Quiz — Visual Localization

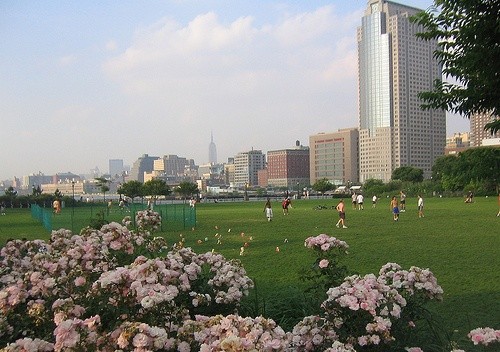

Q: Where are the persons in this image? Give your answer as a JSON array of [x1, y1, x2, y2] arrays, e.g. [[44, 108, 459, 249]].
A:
[[357, 193, 363, 210], [352, 192, 357, 208], [53, 200, 60, 212], [418, 195, 424, 218], [282, 198, 292, 216], [391, 196, 399, 222], [108, 199, 112, 208], [119, 198, 130, 212], [372, 194, 377, 208], [263, 198, 273, 221], [336, 199, 347, 228], [400, 190, 406, 212], [465, 191, 473, 203]]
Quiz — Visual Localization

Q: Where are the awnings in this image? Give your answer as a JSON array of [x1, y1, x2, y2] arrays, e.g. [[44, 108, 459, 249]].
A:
[[338, 186, 346, 189], [350, 186, 361, 189]]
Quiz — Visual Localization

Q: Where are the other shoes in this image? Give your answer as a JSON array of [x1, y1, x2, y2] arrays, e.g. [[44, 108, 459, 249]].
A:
[[336, 224, 339, 228], [343, 225, 348, 229]]
